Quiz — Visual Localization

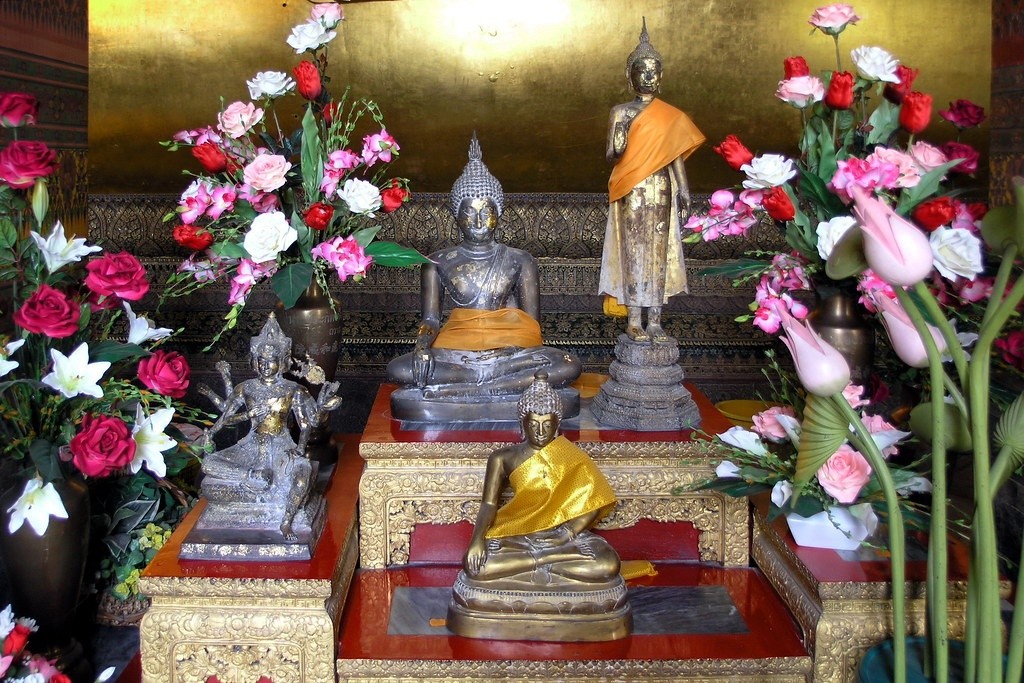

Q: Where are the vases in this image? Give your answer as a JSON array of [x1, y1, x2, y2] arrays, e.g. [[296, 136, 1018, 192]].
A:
[[5, 91, 225, 683], [172, 3, 447, 449], [676, 4, 995, 398]]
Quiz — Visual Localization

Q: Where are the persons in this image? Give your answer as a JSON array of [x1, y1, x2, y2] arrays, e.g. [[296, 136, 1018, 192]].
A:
[[461, 365, 624, 589], [196, 308, 342, 542], [383, 128, 582, 400], [597, 16, 706, 344]]
